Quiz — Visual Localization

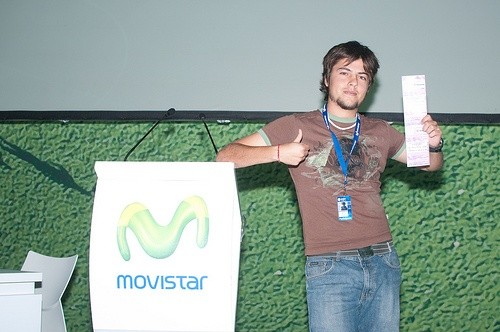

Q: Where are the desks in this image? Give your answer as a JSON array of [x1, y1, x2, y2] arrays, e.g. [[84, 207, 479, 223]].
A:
[[0, 269, 43, 332]]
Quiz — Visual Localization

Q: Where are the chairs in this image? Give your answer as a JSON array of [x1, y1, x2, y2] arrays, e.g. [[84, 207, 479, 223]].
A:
[[21, 251, 78, 332]]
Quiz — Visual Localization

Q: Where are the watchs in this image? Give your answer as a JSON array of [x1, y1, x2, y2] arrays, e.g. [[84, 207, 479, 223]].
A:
[[428, 138, 443, 152]]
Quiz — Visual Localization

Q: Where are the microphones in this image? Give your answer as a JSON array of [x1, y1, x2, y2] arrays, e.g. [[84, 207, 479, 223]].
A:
[[124, 108, 175, 161], [199, 113, 218, 155]]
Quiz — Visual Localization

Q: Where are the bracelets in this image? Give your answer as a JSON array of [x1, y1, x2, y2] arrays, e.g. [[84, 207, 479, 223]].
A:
[[278, 144, 280, 162]]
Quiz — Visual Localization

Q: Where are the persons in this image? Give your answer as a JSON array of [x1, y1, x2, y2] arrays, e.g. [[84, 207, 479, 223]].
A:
[[215, 40, 444, 331]]
